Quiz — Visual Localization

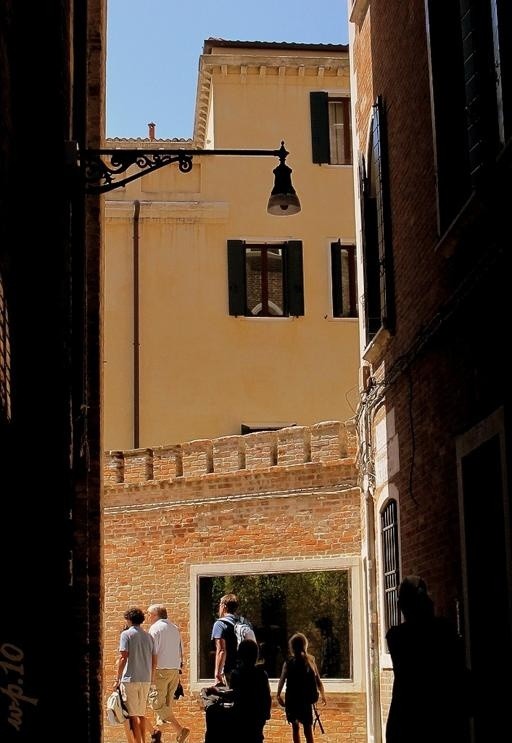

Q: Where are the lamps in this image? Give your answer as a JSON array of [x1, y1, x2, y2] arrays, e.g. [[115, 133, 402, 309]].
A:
[[73, 140, 301, 217]]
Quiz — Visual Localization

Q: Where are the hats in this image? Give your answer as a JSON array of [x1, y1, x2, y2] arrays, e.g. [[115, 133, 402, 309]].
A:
[[398, 574, 428, 599]]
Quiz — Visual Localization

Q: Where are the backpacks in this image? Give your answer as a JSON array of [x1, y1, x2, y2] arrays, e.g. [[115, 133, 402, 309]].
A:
[[285, 655, 319, 707], [215, 614, 259, 659]]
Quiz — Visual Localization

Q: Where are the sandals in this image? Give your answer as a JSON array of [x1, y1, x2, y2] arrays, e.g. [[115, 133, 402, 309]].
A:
[[150, 729, 162, 743], [175, 726, 191, 743]]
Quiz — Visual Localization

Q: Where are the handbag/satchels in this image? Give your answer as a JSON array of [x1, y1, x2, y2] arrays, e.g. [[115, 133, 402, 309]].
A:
[[105, 690, 129, 726], [173, 683, 184, 700]]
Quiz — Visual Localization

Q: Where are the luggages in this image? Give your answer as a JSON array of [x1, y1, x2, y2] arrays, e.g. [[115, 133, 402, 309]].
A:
[[203, 687, 257, 743]]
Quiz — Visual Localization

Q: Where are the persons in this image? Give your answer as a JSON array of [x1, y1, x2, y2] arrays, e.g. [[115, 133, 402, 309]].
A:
[[384, 574, 472, 743], [209, 592, 255, 688], [144, 602, 191, 742], [275, 632, 329, 743], [250, 622, 276, 678], [204, 638, 274, 743], [313, 615, 342, 678], [115, 622, 143, 743], [112, 606, 158, 743], [269, 623, 286, 678]]
